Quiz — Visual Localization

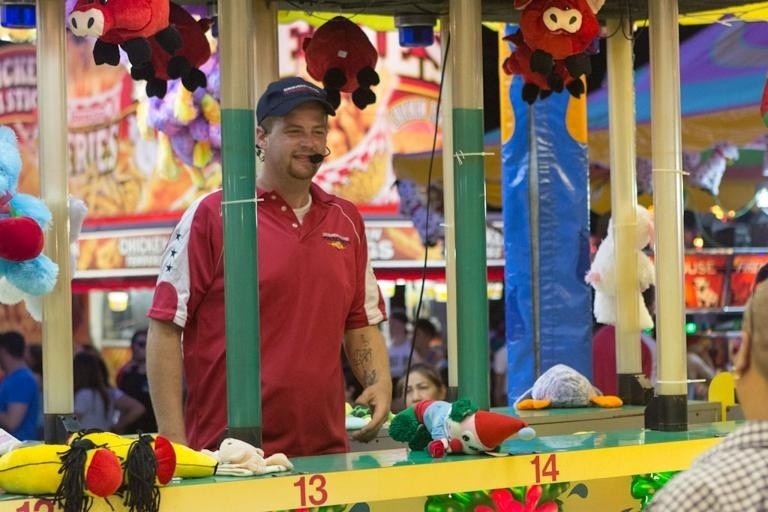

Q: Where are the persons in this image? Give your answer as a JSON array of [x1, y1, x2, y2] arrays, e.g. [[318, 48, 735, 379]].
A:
[[144, 77, 393, 457], [646, 284, 768, 512], [593, 284, 657, 400], [70, 352, 145, 436], [493, 348, 508, 407], [117, 330, 158, 435], [344, 366, 365, 405], [397, 364, 447, 410], [387, 311, 443, 379], [0, 331, 46, 441]]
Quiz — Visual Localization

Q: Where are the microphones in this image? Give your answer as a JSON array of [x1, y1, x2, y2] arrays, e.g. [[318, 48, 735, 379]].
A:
[[310, 146, 331, 164]]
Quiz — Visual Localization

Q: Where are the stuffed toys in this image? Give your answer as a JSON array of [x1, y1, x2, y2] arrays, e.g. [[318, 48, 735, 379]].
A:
[[584, 203, 654, 328], [0, 127, 58, 296], [147, 53, 221, 169], [394, 179, 445, 246], [388, 397, 536, 458], [66, 0, 214, 98], [0, 430, 294, 512], [512, 364, 624, 411], [636, 144, 742, 196], [502, 0, 606, 106], [302, 16, 381, 111]]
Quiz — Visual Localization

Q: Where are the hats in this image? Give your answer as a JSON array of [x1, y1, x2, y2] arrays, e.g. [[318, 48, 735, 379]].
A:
[[257, 77, 335, 125]]
